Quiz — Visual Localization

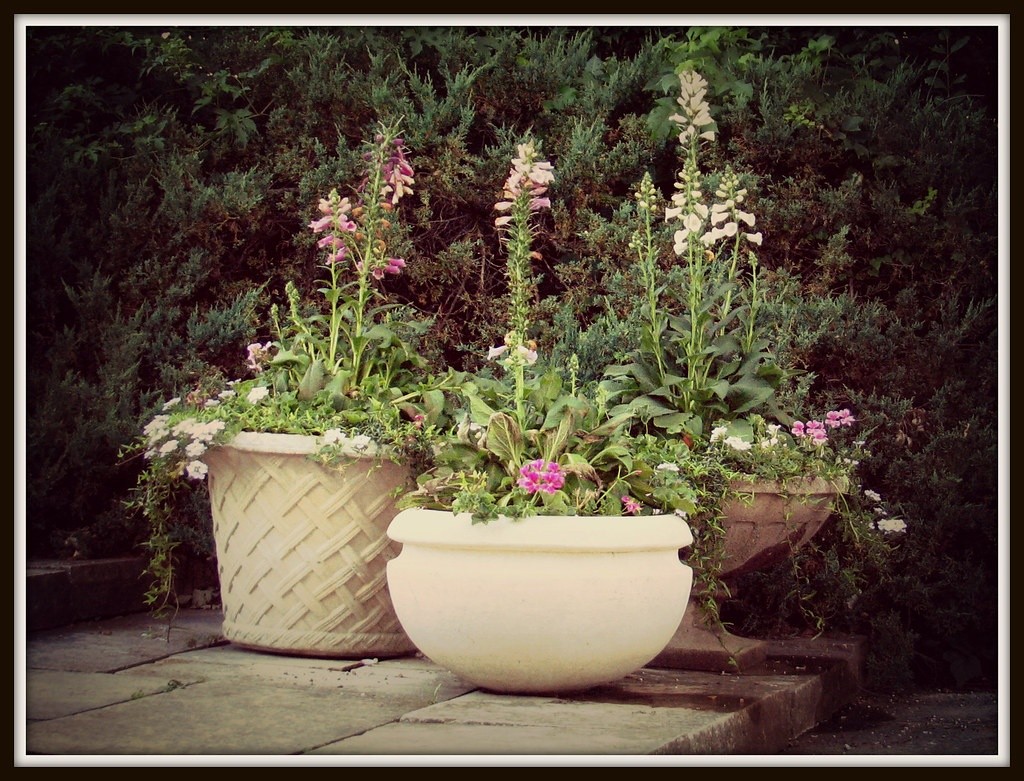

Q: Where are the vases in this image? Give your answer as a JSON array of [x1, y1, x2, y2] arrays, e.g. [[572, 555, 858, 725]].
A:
[[386, 509, 694, 694], [203, 430, 421, 656], [655, 473, 852, 670]]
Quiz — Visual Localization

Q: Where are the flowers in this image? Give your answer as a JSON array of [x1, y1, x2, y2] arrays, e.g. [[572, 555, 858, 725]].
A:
[[117, 62, 912, 647]]
